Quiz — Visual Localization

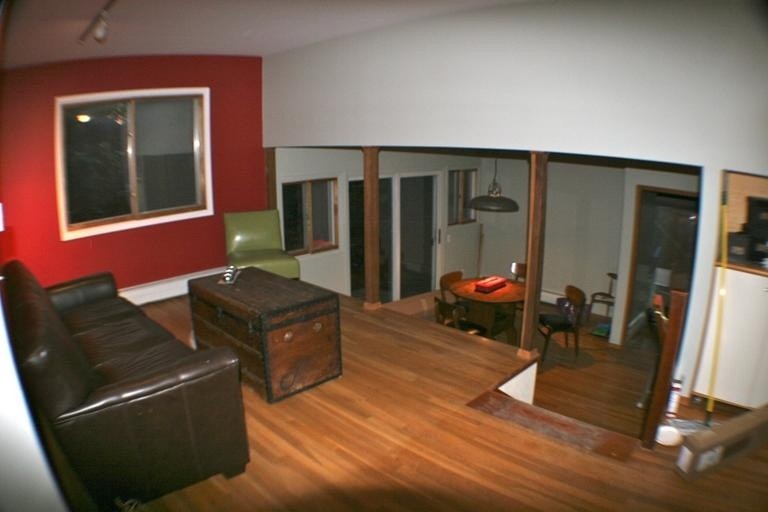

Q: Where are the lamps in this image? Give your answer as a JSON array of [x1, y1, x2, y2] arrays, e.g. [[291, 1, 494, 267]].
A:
[[653, 268, 672, 295], [466, 157, 520, 213]]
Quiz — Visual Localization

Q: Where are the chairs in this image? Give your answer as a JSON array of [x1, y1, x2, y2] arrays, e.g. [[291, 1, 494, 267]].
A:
[[223, 209, 300, 281], [433, 262, 585, 367], [586, 272, 618, 329]]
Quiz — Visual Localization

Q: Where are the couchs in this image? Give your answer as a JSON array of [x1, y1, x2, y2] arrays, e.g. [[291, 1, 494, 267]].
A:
[[0, 259, 252, 512]]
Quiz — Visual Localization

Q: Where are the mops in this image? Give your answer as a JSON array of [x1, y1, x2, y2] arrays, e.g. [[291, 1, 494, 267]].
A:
[[666, 188, 729, 438]]
